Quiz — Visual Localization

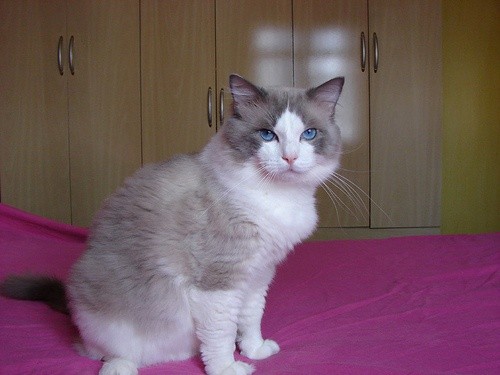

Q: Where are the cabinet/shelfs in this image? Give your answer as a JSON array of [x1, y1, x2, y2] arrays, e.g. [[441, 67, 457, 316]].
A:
[[0, 0, 443, 242]]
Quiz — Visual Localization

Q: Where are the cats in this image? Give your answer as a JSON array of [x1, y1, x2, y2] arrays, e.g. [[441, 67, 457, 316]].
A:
[[1, 70, 395, 375]]
